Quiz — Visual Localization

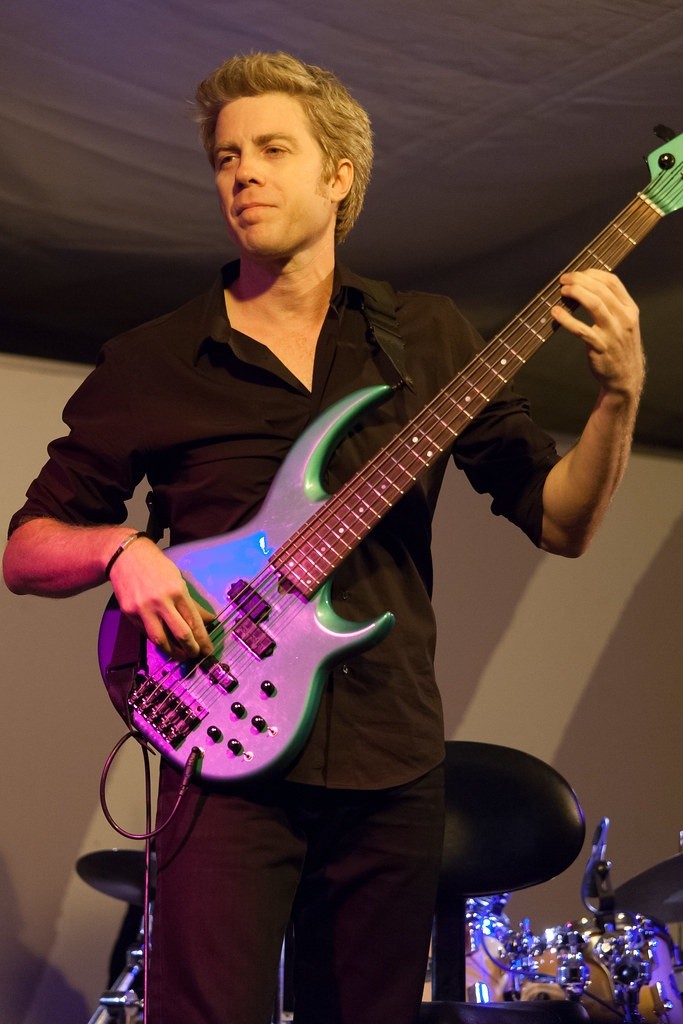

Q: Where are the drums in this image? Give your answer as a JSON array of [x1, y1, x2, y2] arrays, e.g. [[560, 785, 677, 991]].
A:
[[420, 910, 522, 1003], [577, 911, 683, 1024], [513, 924, 638, 1015]]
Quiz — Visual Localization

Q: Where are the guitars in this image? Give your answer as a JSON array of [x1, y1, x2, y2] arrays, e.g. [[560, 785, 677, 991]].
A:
[[93, 129, 683, 787]]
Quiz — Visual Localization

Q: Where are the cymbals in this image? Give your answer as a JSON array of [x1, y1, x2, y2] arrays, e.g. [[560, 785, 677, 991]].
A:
[[596, 852, 683, 923], [437, 741, 586, 898], [75, 850, 156, 907]]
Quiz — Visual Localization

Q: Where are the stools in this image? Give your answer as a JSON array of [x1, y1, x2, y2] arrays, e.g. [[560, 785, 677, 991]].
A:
[[413, 739, 591, 1024]]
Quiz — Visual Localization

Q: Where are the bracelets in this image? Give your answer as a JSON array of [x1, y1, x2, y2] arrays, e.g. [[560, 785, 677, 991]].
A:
[[105, 531, 152, 581]]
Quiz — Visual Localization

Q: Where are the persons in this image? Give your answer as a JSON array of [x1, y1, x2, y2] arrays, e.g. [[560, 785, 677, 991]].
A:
[[4, 54, 645, 1024]]
